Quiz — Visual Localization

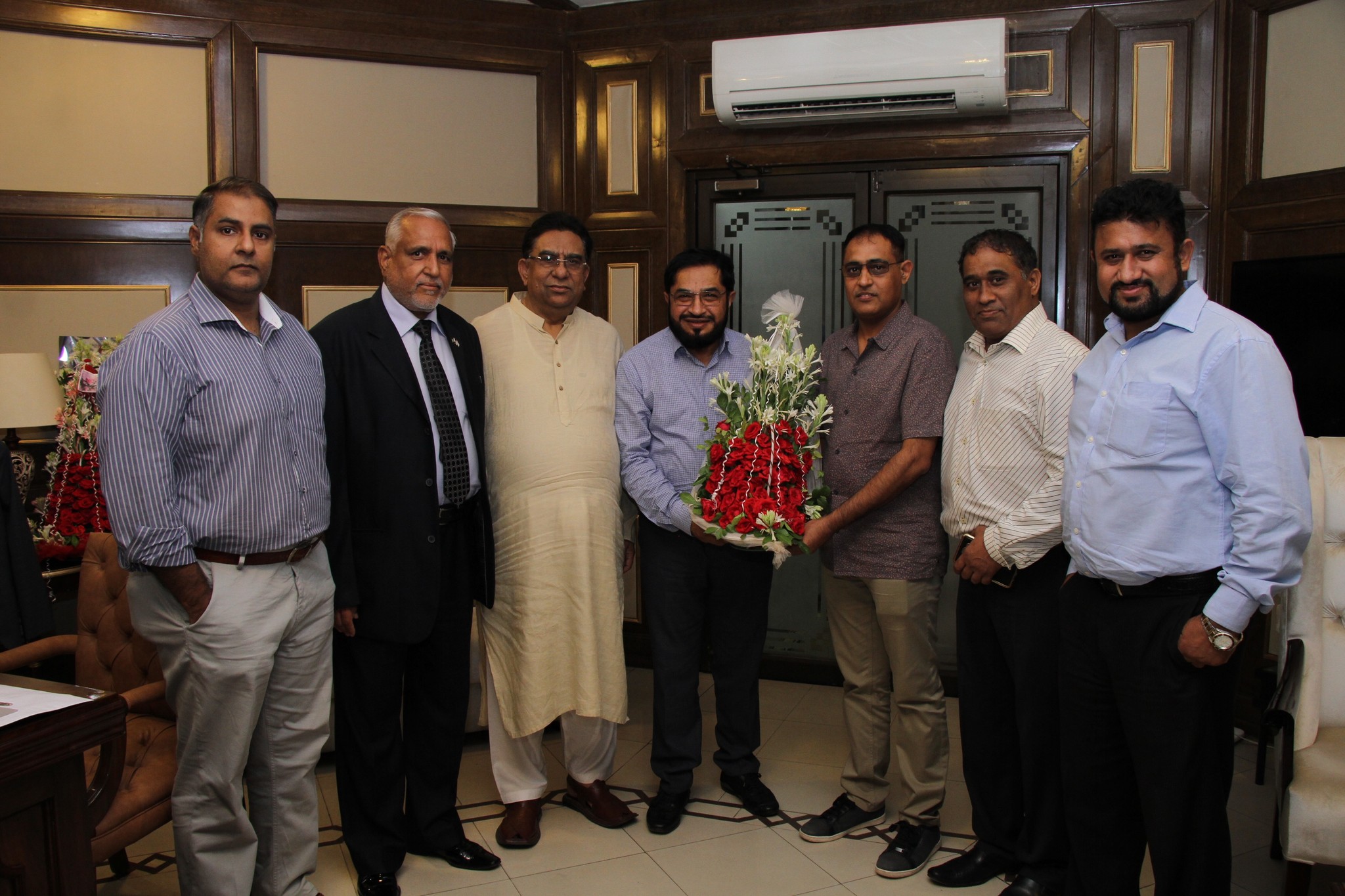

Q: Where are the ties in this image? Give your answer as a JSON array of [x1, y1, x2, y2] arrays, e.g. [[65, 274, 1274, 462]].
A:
[[412, 320, 471, 508]]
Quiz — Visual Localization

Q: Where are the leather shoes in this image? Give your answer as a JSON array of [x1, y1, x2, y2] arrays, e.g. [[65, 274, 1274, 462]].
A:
[[357, 867, 401, 896], [563, 773, 639, 828], [496, 797, 542, 845], [927, 841, 1006, 887], [646, 779, 691, 834], [438, 839, 501, 871], [997, 873, 1045, 896], [720, 771, 779, 817]]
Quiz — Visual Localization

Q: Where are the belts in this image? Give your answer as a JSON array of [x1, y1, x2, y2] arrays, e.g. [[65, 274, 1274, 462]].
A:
[[439, 499, 480, 526], [1092, 569, 1221, 600], [192, 533, 323, 565]]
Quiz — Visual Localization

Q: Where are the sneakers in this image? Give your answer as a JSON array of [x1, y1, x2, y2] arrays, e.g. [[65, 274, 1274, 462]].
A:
[[875, 820, 942, 878], [799, 792, 886, 843]]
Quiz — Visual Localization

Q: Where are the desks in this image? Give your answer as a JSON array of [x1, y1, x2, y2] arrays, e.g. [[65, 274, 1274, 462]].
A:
[[0, 671, 121, 896]]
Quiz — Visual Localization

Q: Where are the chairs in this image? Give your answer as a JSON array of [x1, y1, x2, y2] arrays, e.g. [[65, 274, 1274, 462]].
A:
[[0, 532, 177, 881], [1262, 435, 1344, 896]]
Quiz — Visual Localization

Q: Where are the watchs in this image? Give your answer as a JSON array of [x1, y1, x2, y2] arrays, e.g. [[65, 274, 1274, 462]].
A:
[[1198, 610, 1243, 652]]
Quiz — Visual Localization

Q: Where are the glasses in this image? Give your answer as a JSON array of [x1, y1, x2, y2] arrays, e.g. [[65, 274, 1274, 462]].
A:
[[670, 291, 727, 305], [528, 254, 588, 270], [840, 260, 901, 278]]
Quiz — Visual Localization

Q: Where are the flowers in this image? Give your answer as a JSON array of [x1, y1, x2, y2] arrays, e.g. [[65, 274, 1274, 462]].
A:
[[677, 289, 835, 569], [23, 333, 126, 559]]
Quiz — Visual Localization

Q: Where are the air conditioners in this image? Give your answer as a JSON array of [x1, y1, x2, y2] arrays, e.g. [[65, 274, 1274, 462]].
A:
[[712, 18, 1010, 128]]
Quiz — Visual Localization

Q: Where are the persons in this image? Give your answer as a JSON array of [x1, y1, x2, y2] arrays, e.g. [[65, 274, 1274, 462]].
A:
[[293, 210, 503, 896], [615, 244, 788, 834], [1053, 178, 1315, 896], [922, 230, 1091, 896], [788, 223, 956, 879], [95, 176, 338, 896], [465, 213, 638, 850]]
